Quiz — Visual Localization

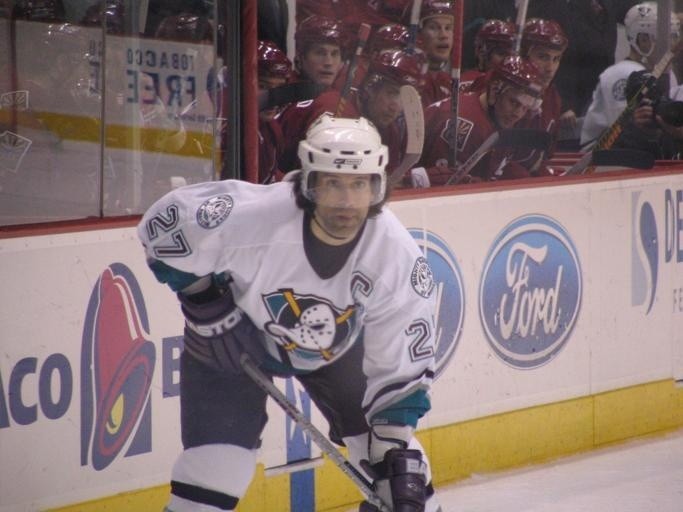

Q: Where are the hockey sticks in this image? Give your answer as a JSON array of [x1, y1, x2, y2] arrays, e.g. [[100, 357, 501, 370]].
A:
[[561, 151, 656, 178], [447, 129, 556, 183]]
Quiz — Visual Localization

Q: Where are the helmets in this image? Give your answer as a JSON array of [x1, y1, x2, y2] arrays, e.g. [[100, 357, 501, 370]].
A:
[[258, 38, 293, 74], [494, 57, 545, 99], [18, 0, 209, 121], [624, 0, 683, 57], [363, 50, 420, 88], [360, 22, 411, 49], [296, 13, 346, 54], [474, 18, 515, 59], [412, 1, 456, 30], [297, 110, 389, 208], [520, 16, 568, 57]]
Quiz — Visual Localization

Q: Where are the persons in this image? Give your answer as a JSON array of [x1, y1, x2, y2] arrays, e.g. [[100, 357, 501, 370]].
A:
[[134, 110, 443, 511], [0, 1, 682, 225]]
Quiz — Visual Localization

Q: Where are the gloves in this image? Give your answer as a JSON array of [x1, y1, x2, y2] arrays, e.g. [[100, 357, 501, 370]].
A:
[[172, 287, 265, 376], [264, 78, 328, 107], [352, 442, 430, 511]]
